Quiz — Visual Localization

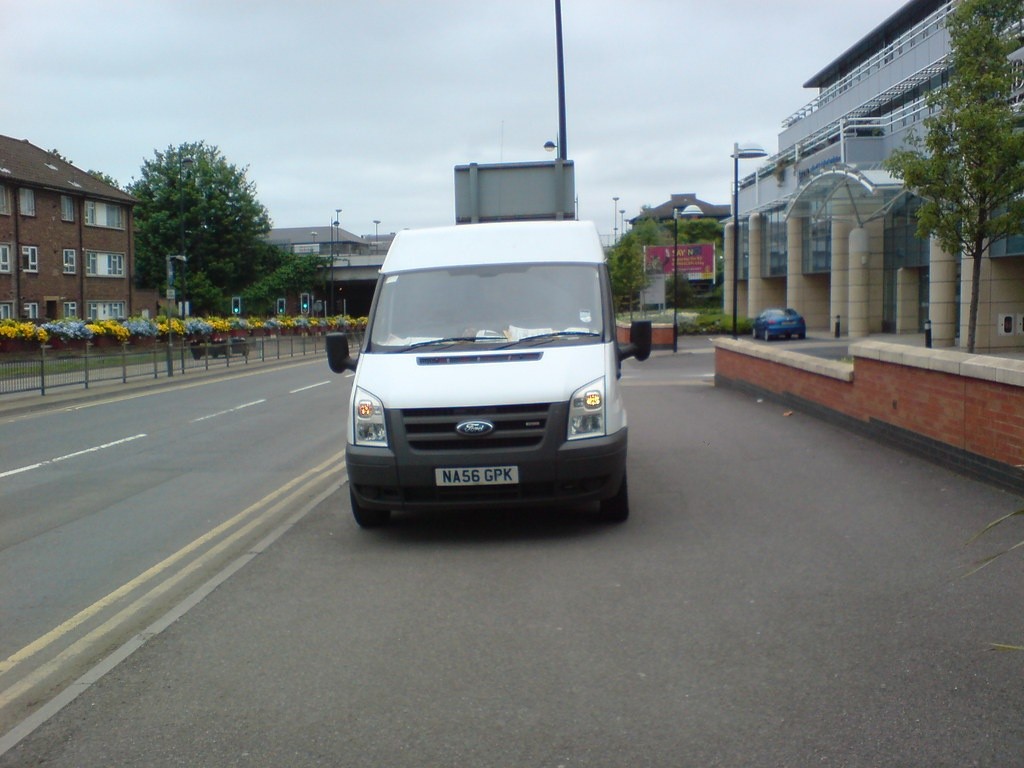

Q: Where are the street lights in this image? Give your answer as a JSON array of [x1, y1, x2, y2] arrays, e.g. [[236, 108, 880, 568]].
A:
[[729, 140, 768, 338], [330, 217, 340, 316], [612, 196, 619, 245], [166, 253, 187, 376], [673, 204, 704, 355], [624, 219, 629, 232], [619, 209, 626, 234], [373, 219, 381, 255], [544, 134, 567, 160], [336, 209, 342, 256]]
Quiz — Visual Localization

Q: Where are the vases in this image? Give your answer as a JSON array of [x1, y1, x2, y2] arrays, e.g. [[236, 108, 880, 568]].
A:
[[23, 336, 42, 351], [231, 328, 249, 337], [160, 332, 178, 343], [320, 326, 330, 333], [94, 334, 124, 347], [210, 332, 228, 344], [280, 326, 295, 336], [188, 331, 203, 341], [336, 325, 345, 332], [296, 327, 311, 337], [50, 334, 90, 350], [266, 328, 278, 335], [251, 327, 266, 336], [127, 333, 157, 348], [309, 327, 321, 335], [0, 337, 24, 353]]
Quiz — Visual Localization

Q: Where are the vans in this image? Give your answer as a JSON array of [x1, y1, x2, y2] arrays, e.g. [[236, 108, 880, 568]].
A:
[[323, 220, 652, 526]]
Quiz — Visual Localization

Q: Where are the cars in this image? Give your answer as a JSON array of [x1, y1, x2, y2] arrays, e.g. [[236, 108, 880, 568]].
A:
[[756, 307, 806, 342]]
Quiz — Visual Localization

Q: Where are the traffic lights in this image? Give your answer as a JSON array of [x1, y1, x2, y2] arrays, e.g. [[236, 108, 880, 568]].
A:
[[299, 292, 310, 315], [277, 299, 286, 315], [232, 296, 240, 315]]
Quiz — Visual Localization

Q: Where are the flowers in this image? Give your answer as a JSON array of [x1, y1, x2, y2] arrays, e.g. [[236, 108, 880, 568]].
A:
[[0, 313, 368, 349]]
